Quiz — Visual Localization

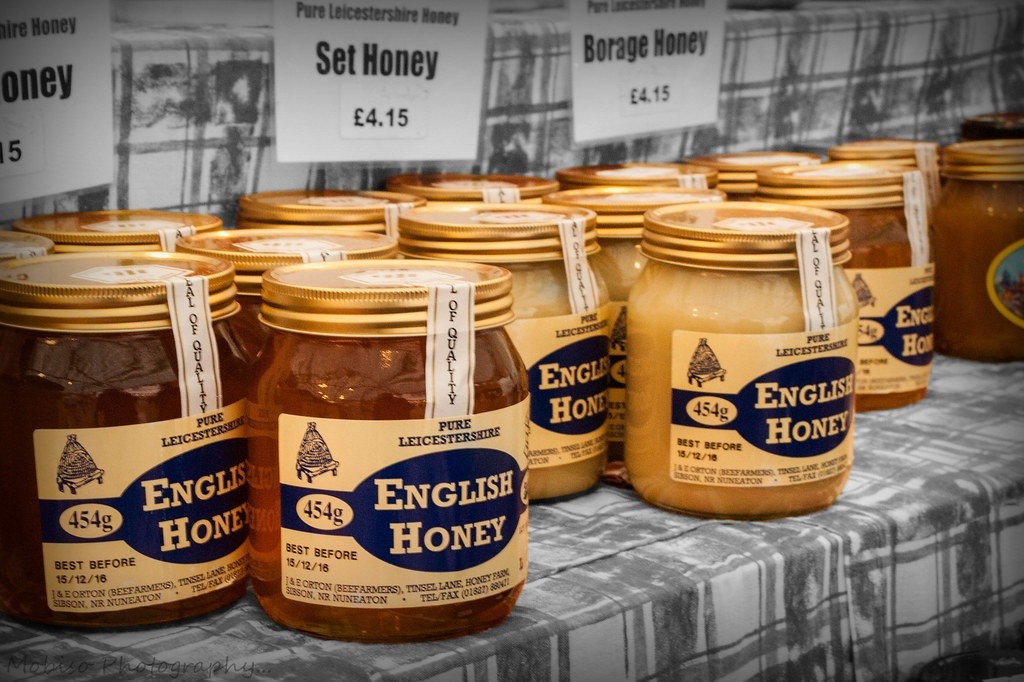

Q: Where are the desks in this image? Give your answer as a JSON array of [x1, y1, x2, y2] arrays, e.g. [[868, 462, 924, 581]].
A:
[[2, 1, 1023, 681]]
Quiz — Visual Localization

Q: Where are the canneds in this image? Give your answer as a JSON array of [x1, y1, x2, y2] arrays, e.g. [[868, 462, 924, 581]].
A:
[[4, 115, 1021, 644]]
